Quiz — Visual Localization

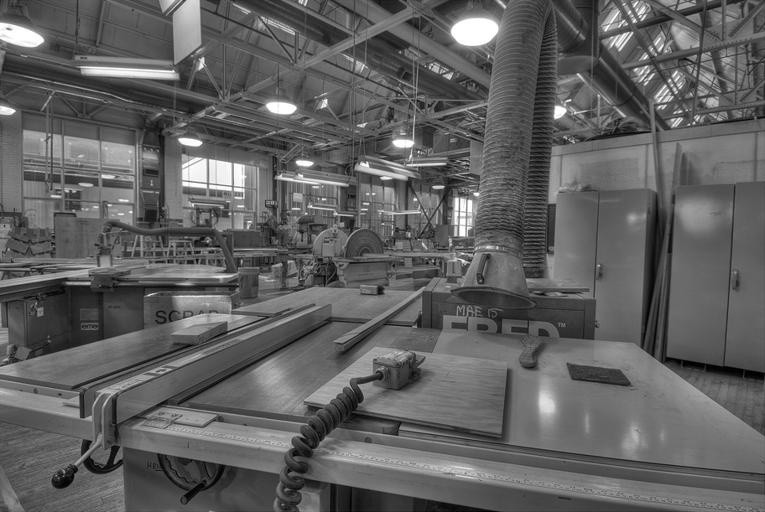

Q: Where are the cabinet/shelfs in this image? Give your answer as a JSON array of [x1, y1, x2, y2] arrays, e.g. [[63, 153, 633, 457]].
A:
[[665, 181, 765, 378], [552, 188, 657, 347]]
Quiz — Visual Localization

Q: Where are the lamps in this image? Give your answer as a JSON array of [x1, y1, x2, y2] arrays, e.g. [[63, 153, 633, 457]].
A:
[[0, 0, 45, 49], [391, 128, 415, 149], [448, 0, 503, 47], [265, 86, 298, 116]]
